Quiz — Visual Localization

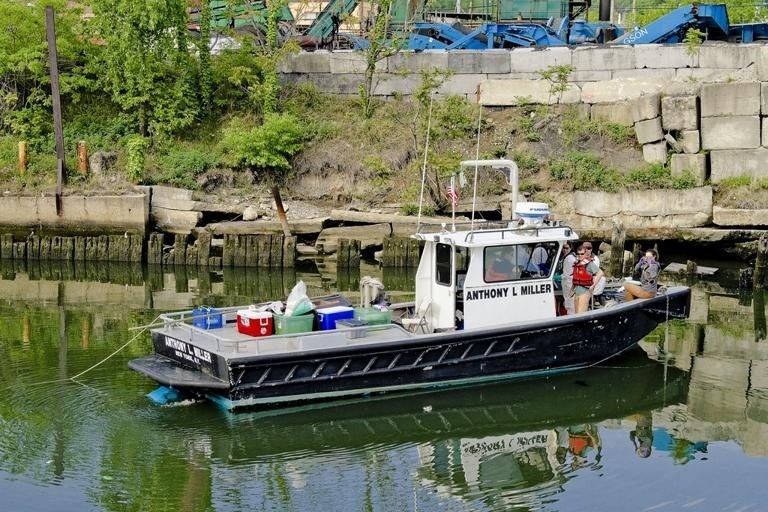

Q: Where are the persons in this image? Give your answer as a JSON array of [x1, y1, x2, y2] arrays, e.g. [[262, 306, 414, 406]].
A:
[[622, 409, 653, 459], [483, 239, 607, 315], [624, 248, 661, 302], [553, 418, 602, 472]]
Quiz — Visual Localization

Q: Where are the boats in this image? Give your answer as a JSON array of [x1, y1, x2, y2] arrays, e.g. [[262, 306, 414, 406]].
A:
[[175, 358, 697, 512], [125, 91, 692, 423]]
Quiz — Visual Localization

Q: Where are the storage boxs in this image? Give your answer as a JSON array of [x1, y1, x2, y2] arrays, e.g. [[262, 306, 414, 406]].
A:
[[236, 305, 354, 337]]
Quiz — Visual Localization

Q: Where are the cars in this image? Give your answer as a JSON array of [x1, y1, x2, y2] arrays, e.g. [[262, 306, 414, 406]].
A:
[[143, 25, 242, 63]]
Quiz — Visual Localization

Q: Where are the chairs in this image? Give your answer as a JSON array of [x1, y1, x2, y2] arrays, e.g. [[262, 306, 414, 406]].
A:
[[402, 295, 433, 334]]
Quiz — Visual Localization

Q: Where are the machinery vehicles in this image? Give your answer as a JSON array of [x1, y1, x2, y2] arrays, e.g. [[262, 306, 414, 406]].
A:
[[186, 0, 361, 49]]
